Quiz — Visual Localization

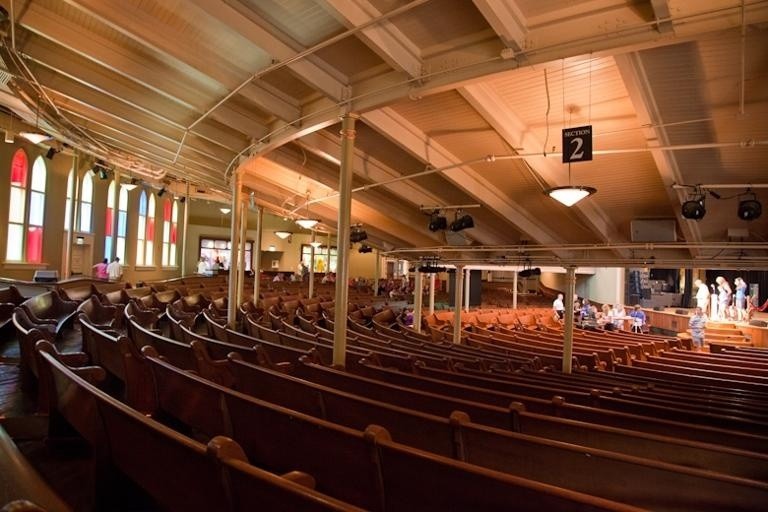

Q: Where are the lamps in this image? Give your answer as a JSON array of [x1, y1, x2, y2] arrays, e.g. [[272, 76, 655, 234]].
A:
[[273, 215, 294, 241], [120, 161, 137, 193], [294, 191, 321, 231], [541, 151, 597, 209], [220, 190, 235, 218], [19, 94, 55, 147]]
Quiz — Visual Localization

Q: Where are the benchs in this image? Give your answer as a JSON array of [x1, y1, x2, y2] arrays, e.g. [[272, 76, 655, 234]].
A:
[[0, 266, 766, 512]]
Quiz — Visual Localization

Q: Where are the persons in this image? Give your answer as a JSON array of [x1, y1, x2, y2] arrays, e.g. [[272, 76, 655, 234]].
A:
[[573, 295, 579, 302], [275, 262, 311, 282], [92, 258, 108, 281], [197, 257, 210, 275], [630, 304, 646, 325], [215, 256, 220, 270], [589, 305, 599, 314], [322, 272, 441, 300], [601, 304, 612, 323], [694, 279, 710, 315], [405, 309, 414, 325], [553, 294, 566, 318], [574, 301, 581, 321], [580, 298, 591, 318], [734, 278, 749, 321], [401, 307, 409, 321], [612, 304, 625, 329], [106, 257, 123, 282], [715, 276, 732, 321], [688, 307, 707, 347]]
